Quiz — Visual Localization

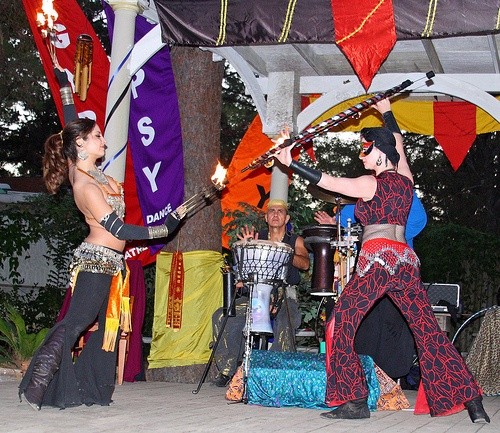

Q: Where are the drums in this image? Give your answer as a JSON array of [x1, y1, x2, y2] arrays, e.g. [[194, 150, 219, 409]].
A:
[[230, 239, 295, 335], [303, 224, 341, 298]]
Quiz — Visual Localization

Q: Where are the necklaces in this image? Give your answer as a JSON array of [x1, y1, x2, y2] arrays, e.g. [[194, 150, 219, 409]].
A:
[[387, 169, 397, 172], [267, 232, 284, 242], [84, 166, 109, 184]]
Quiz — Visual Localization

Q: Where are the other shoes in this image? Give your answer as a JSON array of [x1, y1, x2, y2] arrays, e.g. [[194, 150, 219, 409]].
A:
[[216, 374, 230, 386], [463, 394, 490, 424], [320, 399, 370, 419]]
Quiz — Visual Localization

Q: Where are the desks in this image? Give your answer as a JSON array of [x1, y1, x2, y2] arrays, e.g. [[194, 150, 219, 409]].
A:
[[434, 313, 470, 340]]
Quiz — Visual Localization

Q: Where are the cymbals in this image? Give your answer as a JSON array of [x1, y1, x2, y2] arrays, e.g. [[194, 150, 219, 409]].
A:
[[305, 182, 358, 206]]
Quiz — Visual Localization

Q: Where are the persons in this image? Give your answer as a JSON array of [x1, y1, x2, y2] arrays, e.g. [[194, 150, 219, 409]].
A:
[[18, 42, 187, 412], [212, 199, 310, 387], [314, 189, 427, 380], [267, 93, 490, 424]]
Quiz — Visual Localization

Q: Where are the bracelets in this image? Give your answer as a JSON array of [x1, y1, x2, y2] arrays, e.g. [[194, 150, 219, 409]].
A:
[[147, 223, 168, 239]]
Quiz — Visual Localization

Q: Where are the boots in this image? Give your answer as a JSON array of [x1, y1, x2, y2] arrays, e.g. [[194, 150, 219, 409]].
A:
[[23, 325, 65, 411]]
